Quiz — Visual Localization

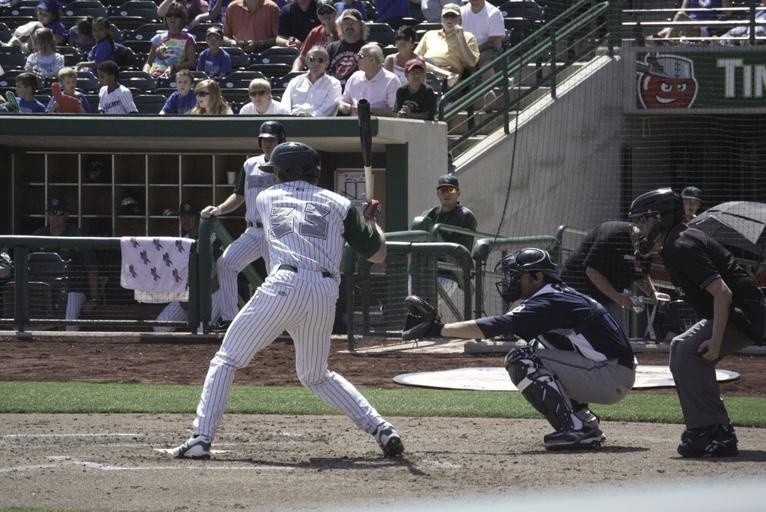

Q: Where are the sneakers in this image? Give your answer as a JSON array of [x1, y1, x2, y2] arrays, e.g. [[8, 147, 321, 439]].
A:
[[171, 436, 211, 459], [678, 422, 738, 457], [208, 316, 231, 332], [544, 407, 606, 451], [374, 421, 404, 456]]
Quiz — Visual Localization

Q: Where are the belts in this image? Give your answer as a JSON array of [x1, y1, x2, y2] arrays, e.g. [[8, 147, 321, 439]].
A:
[[277, 263, 332, 277], [245, 222, 262, 227]]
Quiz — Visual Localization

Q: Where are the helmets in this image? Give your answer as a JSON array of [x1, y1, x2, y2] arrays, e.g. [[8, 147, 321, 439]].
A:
[[258, 121, 285, 143], [495, 248, 558, 301], [626, 187, 684, 259], [259, 142, 321, 183]]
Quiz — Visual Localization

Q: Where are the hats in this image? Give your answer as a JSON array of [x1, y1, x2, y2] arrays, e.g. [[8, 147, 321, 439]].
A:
[[179, 202, 200, 217], [317, 0, 336, 15], [47, 199, 67, 212], [442, 4, 460, 17], [436, 175, 458, 190], [405, 60, 425, 73], [682, 185, 703, 202], [206, 26, 222, 38]]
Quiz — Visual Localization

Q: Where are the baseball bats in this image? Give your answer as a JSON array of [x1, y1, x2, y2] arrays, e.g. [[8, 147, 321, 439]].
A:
[[357, 99, 374, 202]]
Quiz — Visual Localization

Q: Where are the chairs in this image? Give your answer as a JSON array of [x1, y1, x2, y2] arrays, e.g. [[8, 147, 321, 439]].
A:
[[0, 1, 549, 115]]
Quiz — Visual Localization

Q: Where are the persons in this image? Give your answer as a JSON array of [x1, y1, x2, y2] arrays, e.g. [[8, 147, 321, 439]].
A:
[[407, 175, 478, 306], [402, 247, 639, 449], [643, 0, 766, 46], [171, 141, 404, 457], [0, 196, 225, 331], [200, 120, 285, 333], [0, 0, 547, 120], [560, 221, 656, 310], [654, 185, 705, 344], [628, 187, 766, 456]]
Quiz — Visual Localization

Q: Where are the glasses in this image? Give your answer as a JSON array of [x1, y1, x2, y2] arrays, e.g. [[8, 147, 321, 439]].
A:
[[195, 90, 208, 97], [309, 56, 322, 62], [165, 12, 181, 18], [47, 211, 64, 216], [250, 90, 265, 96]]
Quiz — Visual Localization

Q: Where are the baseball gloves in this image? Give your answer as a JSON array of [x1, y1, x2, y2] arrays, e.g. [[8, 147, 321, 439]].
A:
[[402, 295, 445, 340]]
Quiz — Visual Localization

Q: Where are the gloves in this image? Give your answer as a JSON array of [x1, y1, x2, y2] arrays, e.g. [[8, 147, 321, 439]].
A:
[[361, 200, 381, 221]]
